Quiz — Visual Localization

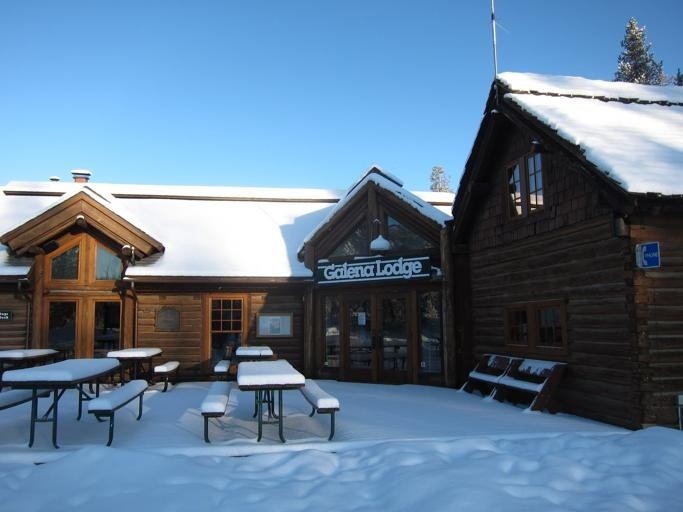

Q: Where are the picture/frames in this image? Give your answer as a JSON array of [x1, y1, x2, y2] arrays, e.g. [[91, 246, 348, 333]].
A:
[[255, 312, 294, 338]]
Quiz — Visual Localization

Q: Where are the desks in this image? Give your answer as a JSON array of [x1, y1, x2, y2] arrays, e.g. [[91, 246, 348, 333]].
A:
[[0, 348, 163, 449], [235, 346, 305, 443]]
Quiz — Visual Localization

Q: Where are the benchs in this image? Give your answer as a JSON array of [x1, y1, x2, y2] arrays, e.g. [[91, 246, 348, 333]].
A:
[[454, 353, 568, 415], [300, 378, 341, 440], [88, 362, 182, 446], [201, 359, 232, 443]]
